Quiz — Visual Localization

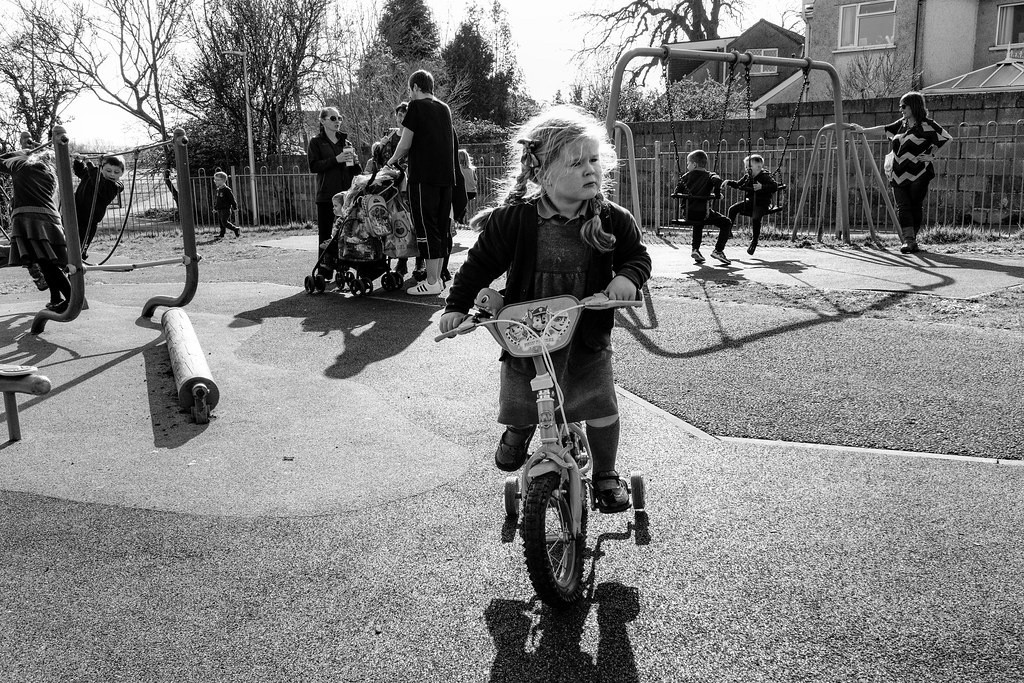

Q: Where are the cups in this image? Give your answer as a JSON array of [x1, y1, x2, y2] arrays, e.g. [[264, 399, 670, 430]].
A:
[[343, 146, 355, 167]]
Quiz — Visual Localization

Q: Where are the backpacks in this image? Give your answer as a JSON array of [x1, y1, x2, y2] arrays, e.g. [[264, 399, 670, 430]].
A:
[[355, 195, 393, 240]]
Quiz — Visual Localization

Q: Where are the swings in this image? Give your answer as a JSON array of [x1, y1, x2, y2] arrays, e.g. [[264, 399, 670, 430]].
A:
[[659, 44, 741, 225], [739, 51, 813, 218]]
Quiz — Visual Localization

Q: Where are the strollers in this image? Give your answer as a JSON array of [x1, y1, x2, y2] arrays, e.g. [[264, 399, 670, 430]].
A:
[[304, 158, 406, 299]]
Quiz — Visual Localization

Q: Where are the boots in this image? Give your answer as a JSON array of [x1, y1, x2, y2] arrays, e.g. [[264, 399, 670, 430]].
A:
[[899, 226, 919, 254]]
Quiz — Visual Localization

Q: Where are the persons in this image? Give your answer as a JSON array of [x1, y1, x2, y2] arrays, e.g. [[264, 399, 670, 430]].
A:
[[0, 149, 70, 314], [438, 103, 654, 513], [306, 107, 362, 280], [721, 154, 778, 255], [317, 191, 348, 250], [385, 68, 457, 296], [212, 172, 241, 239], [673, 149, 733, 265], [379, 101, 426, 276], [456, 149, 478, 199], [849, 92, 953, 253], [27, 152, 126, 291], [363, 141, 381, 175]]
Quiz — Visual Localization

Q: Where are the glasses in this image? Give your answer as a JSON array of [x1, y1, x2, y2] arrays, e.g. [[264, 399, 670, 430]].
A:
[[325, 115, 343, 122], [898, 104, 907, 110]]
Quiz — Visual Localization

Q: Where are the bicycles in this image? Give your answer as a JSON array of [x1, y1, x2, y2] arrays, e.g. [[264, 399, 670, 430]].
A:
[[434, 287, 646, 605]]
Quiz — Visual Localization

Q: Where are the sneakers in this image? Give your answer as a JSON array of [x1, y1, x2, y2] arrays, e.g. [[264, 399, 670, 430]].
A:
[[406, 278, 445, 295]]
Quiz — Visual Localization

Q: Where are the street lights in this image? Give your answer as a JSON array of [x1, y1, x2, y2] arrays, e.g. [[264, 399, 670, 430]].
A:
[[221, 50, 258, 228]]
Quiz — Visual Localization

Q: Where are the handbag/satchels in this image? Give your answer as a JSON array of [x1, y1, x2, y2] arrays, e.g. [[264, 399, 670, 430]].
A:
[[883, 150, 894, 180], [383, 198, 420, 258]]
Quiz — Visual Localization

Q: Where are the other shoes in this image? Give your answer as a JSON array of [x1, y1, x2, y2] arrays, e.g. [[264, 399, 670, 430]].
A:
[[395, 261, 408, 276], [415, 269, 452, 281], [690, 248, 706, 262], [715, 230, 733, 240], [591, 469, 629, 513], [495, 425, 537, 471], [32, 271, 49, 291], [412, 262, 425, 277], [709, 249, 732, 264], [45, 297, 90, 313], [235, 227, 240, 238], [747, 241, 757, 255]]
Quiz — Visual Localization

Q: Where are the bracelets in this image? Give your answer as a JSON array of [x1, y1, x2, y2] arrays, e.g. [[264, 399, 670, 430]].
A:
[[932, 153, 936, 160]]
